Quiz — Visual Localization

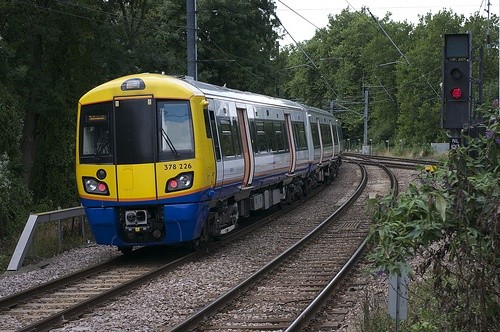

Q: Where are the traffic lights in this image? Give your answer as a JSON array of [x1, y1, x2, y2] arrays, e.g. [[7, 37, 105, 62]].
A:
[[440, 33, 473, 129]]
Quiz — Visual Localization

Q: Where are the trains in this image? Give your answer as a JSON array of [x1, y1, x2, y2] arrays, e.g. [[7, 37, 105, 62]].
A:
[[75, 71, 343, 257]]
